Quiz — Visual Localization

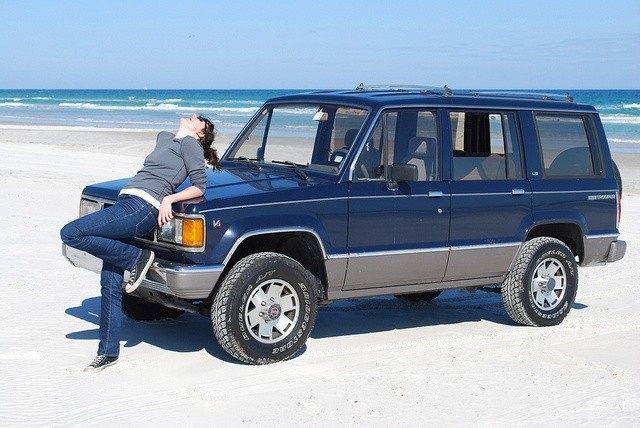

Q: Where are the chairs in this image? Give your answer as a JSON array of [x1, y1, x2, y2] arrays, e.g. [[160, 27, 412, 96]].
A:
[[342, 129, 380, 176], [402, 137, 437, 183]]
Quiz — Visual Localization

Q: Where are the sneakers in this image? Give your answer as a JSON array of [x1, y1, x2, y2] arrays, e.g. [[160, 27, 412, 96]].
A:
[[83, 355, 120, 374], [125, 249, 155, 293]]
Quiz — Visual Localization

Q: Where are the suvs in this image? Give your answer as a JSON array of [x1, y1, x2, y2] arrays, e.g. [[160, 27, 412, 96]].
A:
[[63, 85, 627, 365]]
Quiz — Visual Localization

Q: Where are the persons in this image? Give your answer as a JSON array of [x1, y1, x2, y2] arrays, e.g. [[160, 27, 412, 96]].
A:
[[60, 113, 223, 373]]
